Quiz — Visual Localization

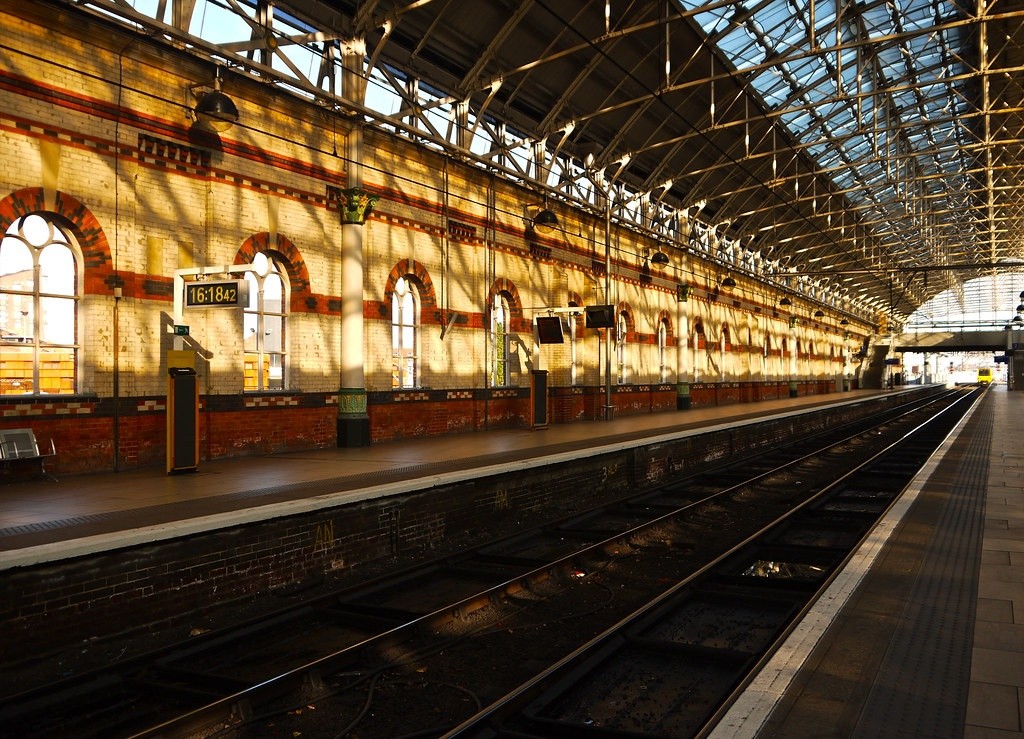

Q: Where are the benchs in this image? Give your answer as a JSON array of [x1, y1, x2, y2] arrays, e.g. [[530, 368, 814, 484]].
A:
[[0, 428, 61, 484]]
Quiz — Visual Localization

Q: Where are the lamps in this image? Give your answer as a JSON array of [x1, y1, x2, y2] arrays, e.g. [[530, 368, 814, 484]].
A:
[[1013, 314, 1023, 323], [776, 289, 792, 308], [836, 314, 850, 327], [1020, 291, 1024, 300], [716, 270, 736, 291], [188, 76, 241, 132], [1016, 303, 1024, 313], [644, 241, 669, 270], [810, 304, 824, 319], [524, 200, 560, 233]]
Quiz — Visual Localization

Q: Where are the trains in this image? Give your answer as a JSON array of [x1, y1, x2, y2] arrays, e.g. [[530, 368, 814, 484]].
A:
[[978, 368, 994, 386]]
